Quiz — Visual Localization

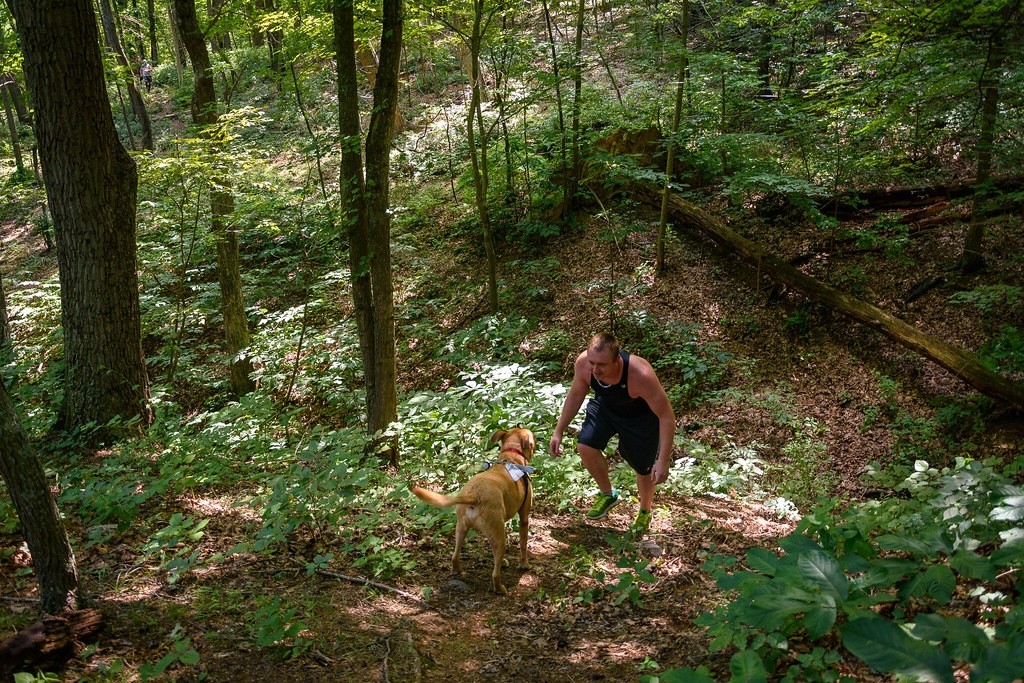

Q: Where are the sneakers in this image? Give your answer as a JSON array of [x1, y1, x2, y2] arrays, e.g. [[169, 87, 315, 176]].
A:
[[630, 509, 652, 536], [586, 488, 620, 520]]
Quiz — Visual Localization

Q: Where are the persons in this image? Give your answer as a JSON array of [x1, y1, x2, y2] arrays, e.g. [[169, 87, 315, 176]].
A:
[[547, 333, 676, 533], [139, 60, 152, 93], [136, 68, 143, 84]]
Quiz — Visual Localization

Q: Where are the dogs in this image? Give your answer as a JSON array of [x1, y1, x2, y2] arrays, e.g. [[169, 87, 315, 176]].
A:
[[411, 428, 534, 595]]
